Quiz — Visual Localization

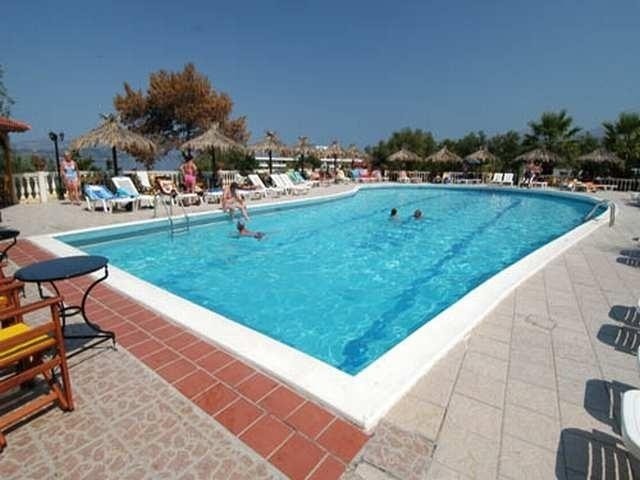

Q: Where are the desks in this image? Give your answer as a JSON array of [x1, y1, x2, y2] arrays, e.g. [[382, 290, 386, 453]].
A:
[[0, 225, 19, 261], [575, 183, 592, 192], [14, 256, 115, 377]]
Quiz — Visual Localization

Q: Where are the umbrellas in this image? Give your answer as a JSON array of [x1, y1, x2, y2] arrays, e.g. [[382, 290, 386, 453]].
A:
[[463, 149, 497, 178], [343, 144, 360, 167], [316, 139, 347, 170], [279, 135, 321, 177], [577, 146, 621, 167], [67, 115, 157, 176], [386, 147, 425, 169], [425, 145, 463, 164], [180, 127, 246, 188], [512, 146, 561, 164], [245, 132, 295, 184]]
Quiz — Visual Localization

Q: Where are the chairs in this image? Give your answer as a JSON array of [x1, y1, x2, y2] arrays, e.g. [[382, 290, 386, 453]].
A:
[[0, 281, 74, 453], [0, 261, 17, 324]]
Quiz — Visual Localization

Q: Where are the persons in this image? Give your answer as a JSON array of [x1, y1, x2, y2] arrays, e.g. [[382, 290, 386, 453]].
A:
[[234, 174, 257, 190], [60, 150, 82, 205], [211, 161, 224, 192], [221, 181, 250, 220], [389, 208, 399, 219], [235, 220, 256, 235], [178, 154, 198, 193], [414, 209, 421, 218]]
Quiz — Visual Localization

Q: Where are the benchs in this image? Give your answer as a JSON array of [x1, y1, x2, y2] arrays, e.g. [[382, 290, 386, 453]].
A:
[[518, 181, 547, 189], [594, 185, 617, 191]]
